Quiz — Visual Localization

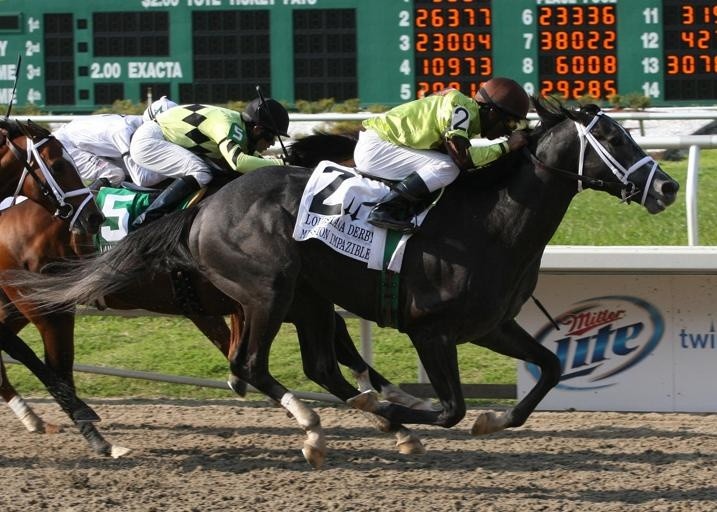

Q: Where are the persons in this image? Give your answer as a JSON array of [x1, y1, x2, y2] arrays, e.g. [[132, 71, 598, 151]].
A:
[[129, 98, 291, 228], [354, 77, 529, 233], [53, 96, 178, 190]]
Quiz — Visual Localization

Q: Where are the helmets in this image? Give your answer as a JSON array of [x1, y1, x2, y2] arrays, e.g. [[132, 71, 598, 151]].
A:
[[475, 78, 533, 124], [143, 96, 179, 124]]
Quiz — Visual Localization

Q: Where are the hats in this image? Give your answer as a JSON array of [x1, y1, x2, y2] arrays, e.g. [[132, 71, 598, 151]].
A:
[[246, 98, 289, 138]]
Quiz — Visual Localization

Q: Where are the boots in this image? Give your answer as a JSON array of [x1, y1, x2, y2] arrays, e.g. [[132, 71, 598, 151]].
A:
[[87, 176, 112, 192], [368, 172, 429, 233], [132, 175, 201, 229]]
